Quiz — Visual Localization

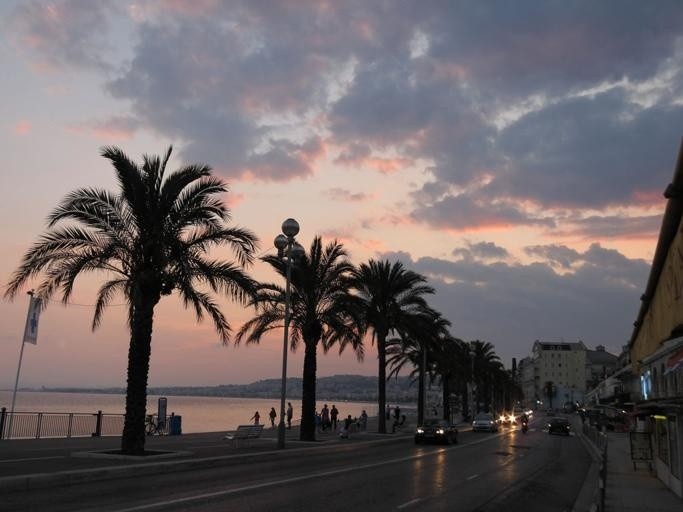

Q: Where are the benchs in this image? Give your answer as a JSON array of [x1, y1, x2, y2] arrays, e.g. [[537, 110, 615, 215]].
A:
[[221, 424, 264, 450]]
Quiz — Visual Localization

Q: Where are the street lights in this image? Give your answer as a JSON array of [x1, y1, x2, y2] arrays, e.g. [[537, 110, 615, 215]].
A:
[[274, 218, 300, 448]]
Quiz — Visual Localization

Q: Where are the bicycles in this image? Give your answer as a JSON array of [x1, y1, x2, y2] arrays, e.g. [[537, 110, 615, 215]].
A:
[[145, 412, 175, 436]]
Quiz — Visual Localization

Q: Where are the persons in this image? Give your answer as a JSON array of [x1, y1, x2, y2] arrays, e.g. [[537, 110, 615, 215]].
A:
[[432, 406, 438, 416], [395, 405, 400, 422], [249, 411, 261, 425], [387, 404, 391, 419], [287, 402, 294, 429], [269, 408, 277, 427], [321, 404, 368, 439], [521, 413, 528, 427]]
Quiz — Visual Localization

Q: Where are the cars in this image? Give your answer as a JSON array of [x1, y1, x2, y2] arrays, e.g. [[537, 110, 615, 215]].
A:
[[415, 417, 457, 445], [472, 414, 499, 434], [547, 416, 570, 436], [497, 404, 534, 426]]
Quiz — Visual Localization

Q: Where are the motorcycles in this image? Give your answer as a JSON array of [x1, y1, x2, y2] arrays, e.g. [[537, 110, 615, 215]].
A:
[[520, 415, 530, 434]]
